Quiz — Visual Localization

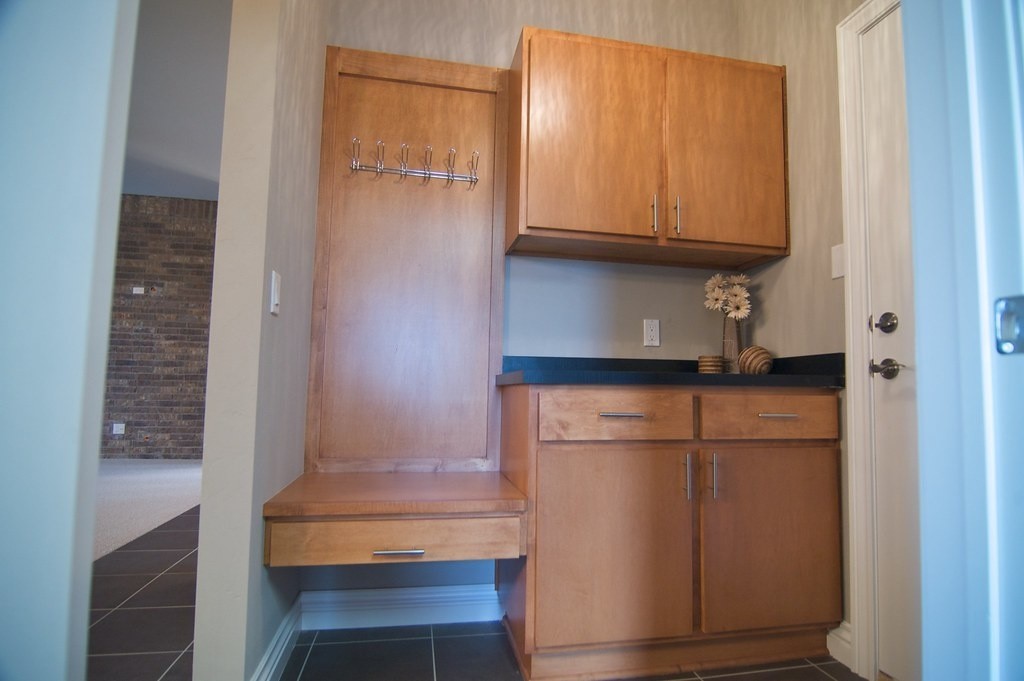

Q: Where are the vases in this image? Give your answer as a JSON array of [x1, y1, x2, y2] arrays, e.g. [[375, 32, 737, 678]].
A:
[[724, 316, 745, 374]]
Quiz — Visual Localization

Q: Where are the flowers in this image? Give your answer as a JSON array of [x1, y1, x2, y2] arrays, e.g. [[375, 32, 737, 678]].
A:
[[703, 273, 752, 320]]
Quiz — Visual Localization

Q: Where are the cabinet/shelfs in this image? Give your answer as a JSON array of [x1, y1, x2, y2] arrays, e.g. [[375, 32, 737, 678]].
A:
[[504, 24, 791, 272], [495, 384, 843, 681], [264, 470, 528, 566]]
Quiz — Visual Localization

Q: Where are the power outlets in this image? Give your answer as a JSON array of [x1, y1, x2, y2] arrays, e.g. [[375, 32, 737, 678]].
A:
[[643, 318, 660, 346]]
